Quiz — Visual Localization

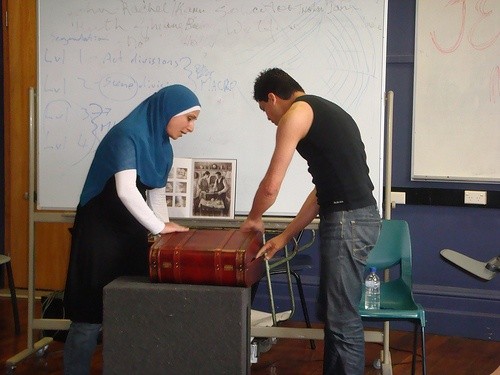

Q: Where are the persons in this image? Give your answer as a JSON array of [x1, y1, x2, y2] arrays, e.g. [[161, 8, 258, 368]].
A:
[[197, 171, 229, 203], [61, 85, 201, 375], [240, 68, 381, 375]]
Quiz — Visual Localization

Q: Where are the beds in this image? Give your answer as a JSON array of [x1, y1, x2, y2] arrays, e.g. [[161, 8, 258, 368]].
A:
[[249, 253, 317, 350]]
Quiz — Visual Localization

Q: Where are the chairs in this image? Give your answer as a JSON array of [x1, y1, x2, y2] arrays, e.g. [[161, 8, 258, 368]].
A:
[[0, 254, 21, 336], [356, 220, 427, 375]]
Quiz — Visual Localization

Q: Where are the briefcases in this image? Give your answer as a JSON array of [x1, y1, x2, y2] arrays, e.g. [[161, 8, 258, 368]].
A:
[[148, 227, 269, 288]]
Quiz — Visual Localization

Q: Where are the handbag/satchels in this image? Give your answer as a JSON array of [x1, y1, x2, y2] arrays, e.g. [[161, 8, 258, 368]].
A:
[[38, 292, 71, 343]]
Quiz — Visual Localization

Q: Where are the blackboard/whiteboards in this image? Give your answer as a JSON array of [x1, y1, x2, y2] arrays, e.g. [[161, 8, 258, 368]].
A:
[[409, 0, 500, 182], [36, 1, 389, 219]]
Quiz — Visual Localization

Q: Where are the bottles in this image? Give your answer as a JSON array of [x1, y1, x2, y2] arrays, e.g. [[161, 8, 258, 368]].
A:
[[364, 266, 381, 310]]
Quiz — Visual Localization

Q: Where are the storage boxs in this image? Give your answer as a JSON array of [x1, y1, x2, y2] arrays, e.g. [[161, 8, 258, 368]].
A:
[[146, 229, 266, 287]]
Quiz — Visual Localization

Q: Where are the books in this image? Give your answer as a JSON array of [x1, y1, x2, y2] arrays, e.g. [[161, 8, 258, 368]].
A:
[[164, 156, 237, 219]]
